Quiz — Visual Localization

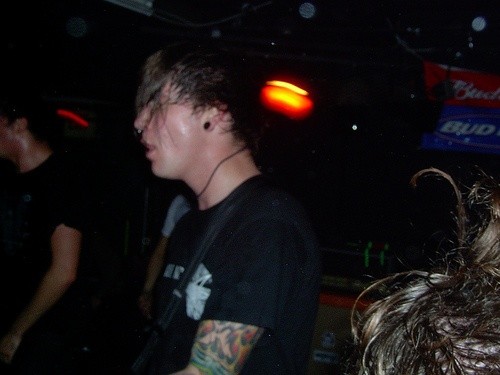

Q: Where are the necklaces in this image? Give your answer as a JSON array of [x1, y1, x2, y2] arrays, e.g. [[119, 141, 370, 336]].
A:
[[197, 144, 250, 198]]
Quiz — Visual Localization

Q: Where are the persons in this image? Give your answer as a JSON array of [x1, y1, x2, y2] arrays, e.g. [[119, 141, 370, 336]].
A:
[[138, 196, 192, 319], [338, 168, 500, 375], [0, 97, 88, 375], [127, 41, 320, 375]]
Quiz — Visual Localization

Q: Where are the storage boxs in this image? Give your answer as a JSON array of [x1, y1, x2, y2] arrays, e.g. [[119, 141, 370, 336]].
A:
[[305, 289, 382, 374]]
